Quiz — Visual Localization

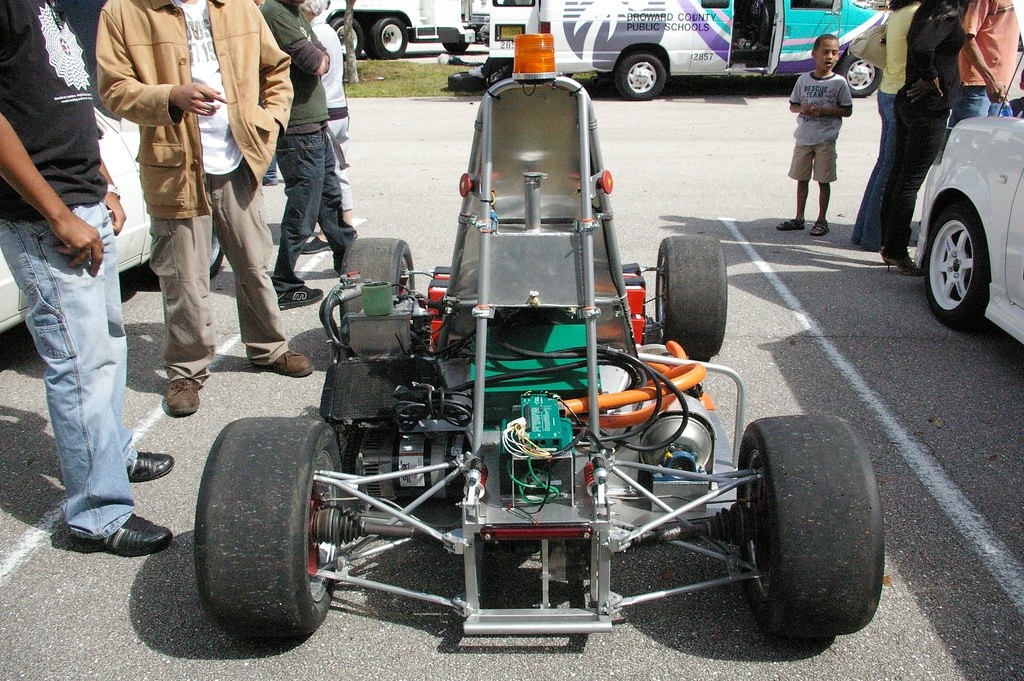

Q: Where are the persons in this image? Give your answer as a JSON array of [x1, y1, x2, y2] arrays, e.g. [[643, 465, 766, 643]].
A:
[[96, 0, 315, 417], [252, 0, 359, 311], [0, 0, 174, 557], [851, 0, 1024, 276], [776, 35, 853, 237]]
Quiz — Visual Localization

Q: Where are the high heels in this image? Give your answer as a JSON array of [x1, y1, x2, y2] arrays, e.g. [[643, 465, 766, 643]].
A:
[[880, 248, 922, 277]]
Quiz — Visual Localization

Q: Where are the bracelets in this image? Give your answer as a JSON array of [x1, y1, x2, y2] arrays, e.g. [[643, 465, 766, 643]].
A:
[[107, 183, 120, 200]]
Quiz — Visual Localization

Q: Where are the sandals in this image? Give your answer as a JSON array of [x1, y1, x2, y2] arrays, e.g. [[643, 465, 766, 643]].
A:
[[809, 219, 829, 236], [776, 219, 805, 231]]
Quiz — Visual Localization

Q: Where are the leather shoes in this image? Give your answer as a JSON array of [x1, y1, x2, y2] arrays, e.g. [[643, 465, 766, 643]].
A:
[[125, 451, 175, 481], [70, 514, 172, 557]]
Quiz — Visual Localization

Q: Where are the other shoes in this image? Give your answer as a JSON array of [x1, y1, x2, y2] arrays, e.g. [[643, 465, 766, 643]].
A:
[[262, 178, 278, 187], [299, 236, 332, 254]]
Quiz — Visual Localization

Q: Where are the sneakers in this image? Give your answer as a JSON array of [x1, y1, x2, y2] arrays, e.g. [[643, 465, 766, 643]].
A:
[[250, 349, 313, 377], [276, 284, 324, 309], [166, 376, 199, 415]]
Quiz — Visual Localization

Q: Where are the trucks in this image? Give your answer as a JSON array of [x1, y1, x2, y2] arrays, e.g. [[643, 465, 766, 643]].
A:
[[319, 0, 490, 61], [489, 0, 894, 101]]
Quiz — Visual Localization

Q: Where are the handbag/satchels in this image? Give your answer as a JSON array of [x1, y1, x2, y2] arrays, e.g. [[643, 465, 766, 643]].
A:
[[847, 23, 888, 69]]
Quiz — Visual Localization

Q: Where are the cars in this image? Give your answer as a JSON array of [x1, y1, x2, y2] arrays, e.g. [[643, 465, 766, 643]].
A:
[[915, 116, 1024, 345], [0, 106, 224, 334]]
